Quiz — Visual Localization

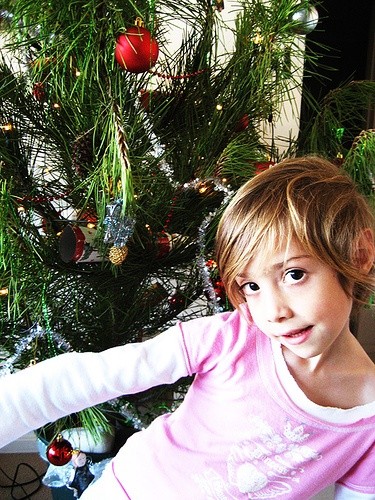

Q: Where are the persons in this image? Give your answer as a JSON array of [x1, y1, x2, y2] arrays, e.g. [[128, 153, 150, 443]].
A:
[[0, 156, 375, 500]]
